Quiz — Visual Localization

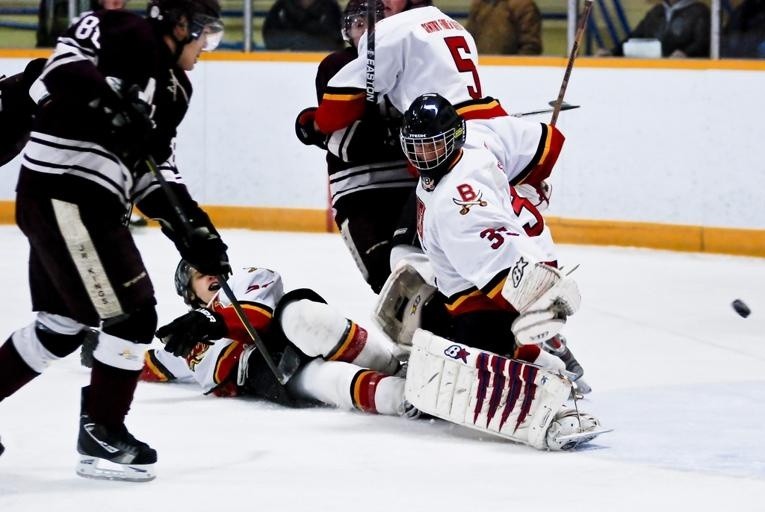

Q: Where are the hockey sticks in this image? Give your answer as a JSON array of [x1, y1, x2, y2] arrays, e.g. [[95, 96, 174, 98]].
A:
[[515, 100, 580, 117], [143, 155, 301, 387]]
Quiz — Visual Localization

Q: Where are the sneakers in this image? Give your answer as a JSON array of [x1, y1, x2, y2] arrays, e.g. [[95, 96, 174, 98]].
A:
[[75, 409, 156, 464], [546, 409, 599, 449], [556, 348, 583, 380]]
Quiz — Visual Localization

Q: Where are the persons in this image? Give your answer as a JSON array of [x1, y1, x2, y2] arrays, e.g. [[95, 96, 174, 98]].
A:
[[0, 0, 229, 481], [261, 0, 345, 53], [79, 255, 450, 421], [596, 0, 765, 59], [36, 0, 125, 50]]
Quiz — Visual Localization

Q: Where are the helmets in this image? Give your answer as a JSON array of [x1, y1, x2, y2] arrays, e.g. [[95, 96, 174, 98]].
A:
[[174, 257, 196, 301], [145, 0, 221, 44], [341, 0, 384, 42], [399, 92, 466, 178]]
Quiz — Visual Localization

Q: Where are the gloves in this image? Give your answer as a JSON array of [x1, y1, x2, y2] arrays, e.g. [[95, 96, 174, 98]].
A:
[[296, 107, 326, 144], [106, 100, 153, 154], [159, 199, 234, 279]]
